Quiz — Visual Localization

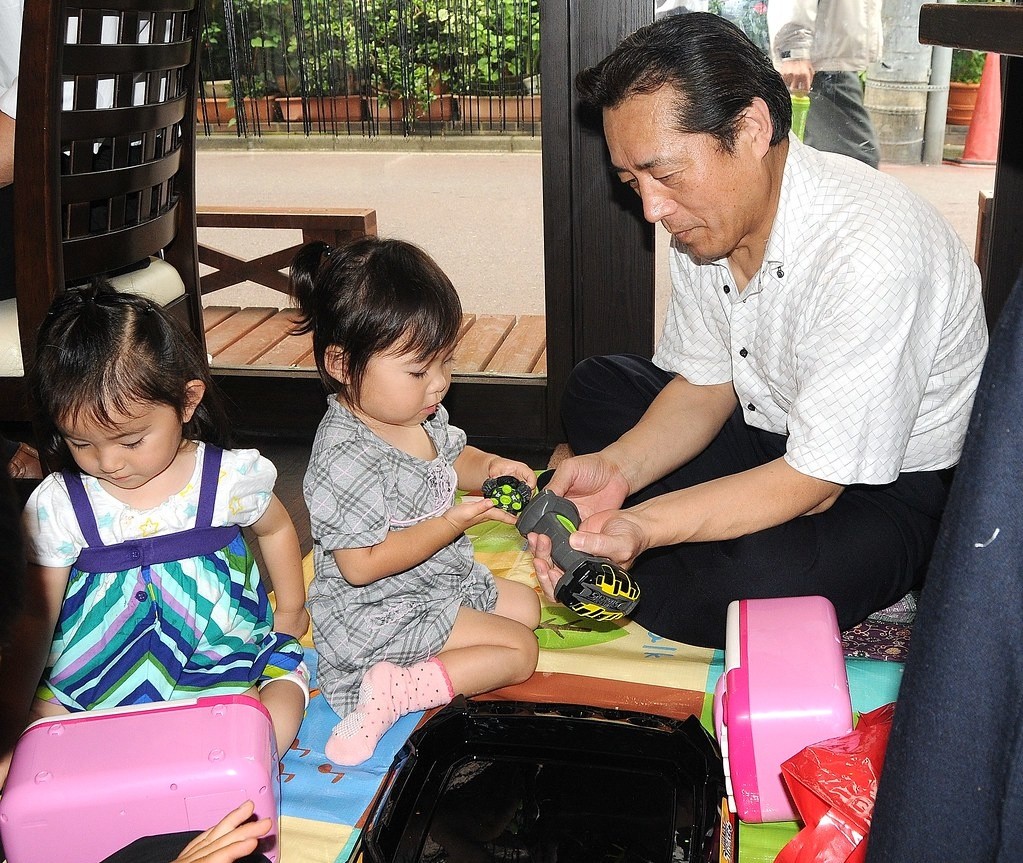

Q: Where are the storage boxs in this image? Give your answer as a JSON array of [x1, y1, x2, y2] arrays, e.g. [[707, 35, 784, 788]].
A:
[[0, 694, 282, 863], [710, 596, 856, 824]]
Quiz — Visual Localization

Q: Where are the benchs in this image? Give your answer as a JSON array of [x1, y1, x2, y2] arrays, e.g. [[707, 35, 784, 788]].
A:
[[195, 203, 379, 302]]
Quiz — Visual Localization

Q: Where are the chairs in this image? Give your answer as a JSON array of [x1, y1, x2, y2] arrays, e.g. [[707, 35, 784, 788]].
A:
[[0, 0, 226, 453]]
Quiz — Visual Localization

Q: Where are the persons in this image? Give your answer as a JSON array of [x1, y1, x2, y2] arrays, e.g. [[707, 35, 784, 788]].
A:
[[18, 274, 311, 763], [0, 483, 276, 863], [765, 0, 887, 171], [522, 9, 992, 649], [1, 0, 178, 304], [284, 237, 544, 767]]
